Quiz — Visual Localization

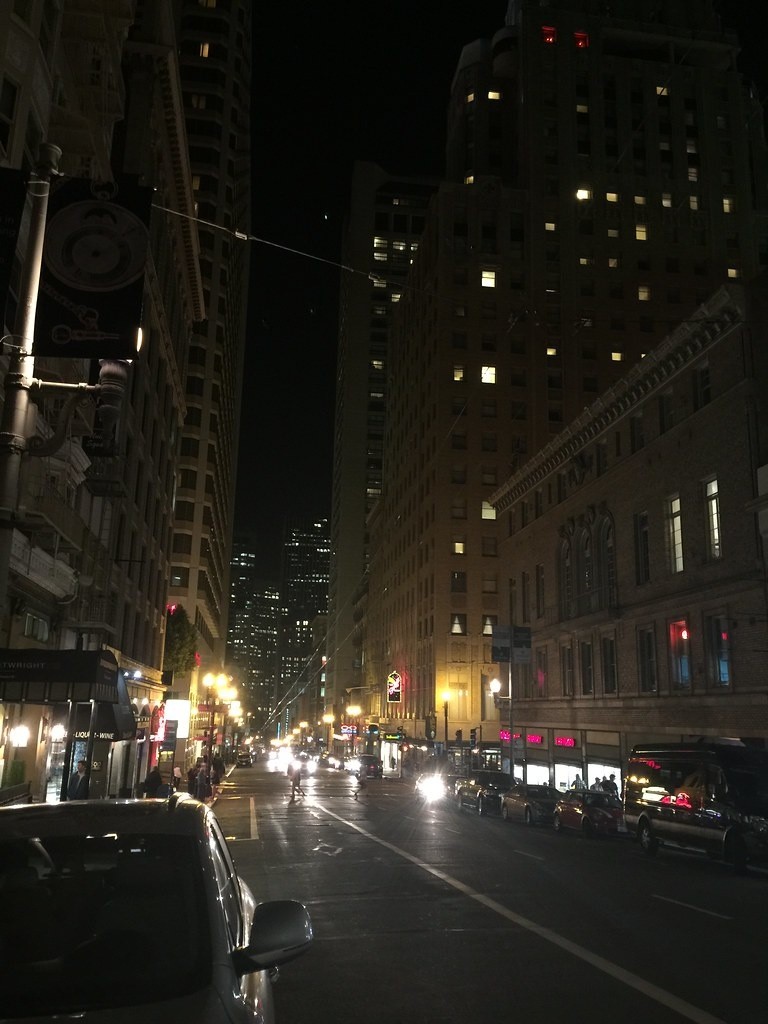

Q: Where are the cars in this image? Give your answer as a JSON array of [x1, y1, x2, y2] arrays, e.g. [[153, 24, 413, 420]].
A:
[[288, 756, 314, 776], [355, 755, 384, 780], [235, 752, 252, 768], [500, 785, 563, 827], [552, 789, 637, 840], [414, 773, 467, 802], [454, 770, 517, 817]]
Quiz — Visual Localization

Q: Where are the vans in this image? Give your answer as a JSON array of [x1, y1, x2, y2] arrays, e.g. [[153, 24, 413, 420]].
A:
[[623, 742, 768, 876]]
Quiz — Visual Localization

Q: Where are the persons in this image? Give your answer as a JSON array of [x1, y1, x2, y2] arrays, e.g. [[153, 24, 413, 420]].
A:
[[351, 766, 368, 800], [571, 774, 619, 800], [286, 761, 306, 796], [145, 765, 162, 798], [67, 760, 90, 801], [187, 749, 232, 802]]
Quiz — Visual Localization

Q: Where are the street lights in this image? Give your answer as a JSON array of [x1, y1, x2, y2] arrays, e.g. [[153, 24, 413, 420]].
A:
[[489, 678, 515, 777], [202, 673, 227, 797], [218, 687, 238, 773], [300, 722, 307, 742], [323, 715, 333, 751], [443, 693, 450, 750], [347, 706, 359, 753]]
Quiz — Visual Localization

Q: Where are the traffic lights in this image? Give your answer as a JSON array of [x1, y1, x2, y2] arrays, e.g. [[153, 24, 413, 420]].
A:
[[456, 730, 461, 746], [397, 726, 403, 739], [401, 744, 408, 752], [471, 729, 476, 750], [426, 716, 436, 740], [372, 726, 378, 735]]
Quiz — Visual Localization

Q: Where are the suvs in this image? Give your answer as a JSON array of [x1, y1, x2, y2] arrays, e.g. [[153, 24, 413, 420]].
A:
[[1, 792, 313, 1024]]
